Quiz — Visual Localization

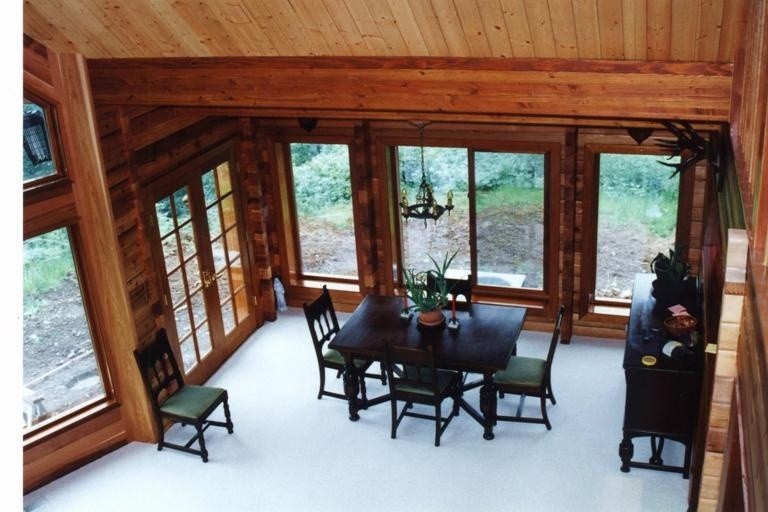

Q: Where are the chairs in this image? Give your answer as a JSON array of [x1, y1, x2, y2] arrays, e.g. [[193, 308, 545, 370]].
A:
[[133, 327, 234, 462], [303, 286, 387, 409], [490, 304, 565, 430], [384, 341, 463, 446], [427, 272, 472, 384]]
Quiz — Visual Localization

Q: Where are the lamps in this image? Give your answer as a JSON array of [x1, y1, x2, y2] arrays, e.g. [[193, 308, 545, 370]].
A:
[[398, 120, 454, 228], [23, 107, 51, 165]]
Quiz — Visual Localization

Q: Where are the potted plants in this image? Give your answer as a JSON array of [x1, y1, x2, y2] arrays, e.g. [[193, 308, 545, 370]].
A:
[[398, 247, 460, 327], [653, 247, 691, 305]]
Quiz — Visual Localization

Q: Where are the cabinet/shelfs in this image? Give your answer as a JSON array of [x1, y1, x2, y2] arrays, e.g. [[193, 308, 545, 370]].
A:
[[619, 272, 702, 480]]
